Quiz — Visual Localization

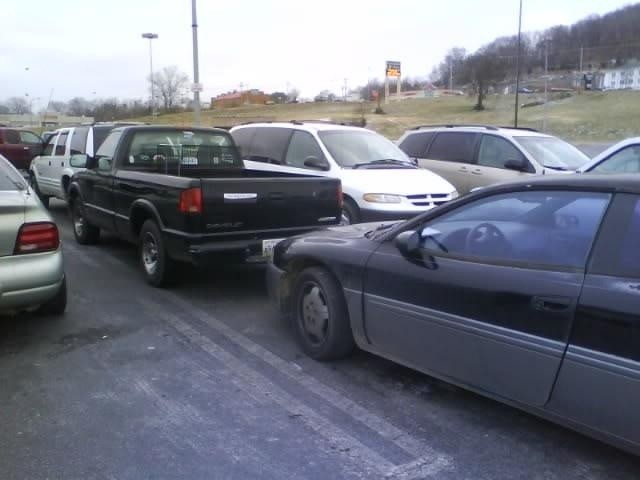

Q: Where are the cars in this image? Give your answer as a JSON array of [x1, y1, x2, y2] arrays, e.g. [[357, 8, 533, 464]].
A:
[[0, 125, 133, 208], [0, 154, 70, 319], [266, 169, 640, 459], [219, 118, 460, 229], [523, 135, 640, 233], [395, 122, 589, 195]]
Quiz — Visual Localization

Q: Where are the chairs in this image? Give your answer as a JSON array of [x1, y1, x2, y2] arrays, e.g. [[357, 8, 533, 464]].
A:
[[140, 142, 223, 165]]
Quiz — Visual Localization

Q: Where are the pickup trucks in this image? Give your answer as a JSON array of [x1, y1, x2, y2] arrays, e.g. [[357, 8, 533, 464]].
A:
[[66, 124, 346, 288]]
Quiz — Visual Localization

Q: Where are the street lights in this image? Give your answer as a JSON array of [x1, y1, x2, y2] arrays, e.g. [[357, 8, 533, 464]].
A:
[[141, 32, 159, 118]]
